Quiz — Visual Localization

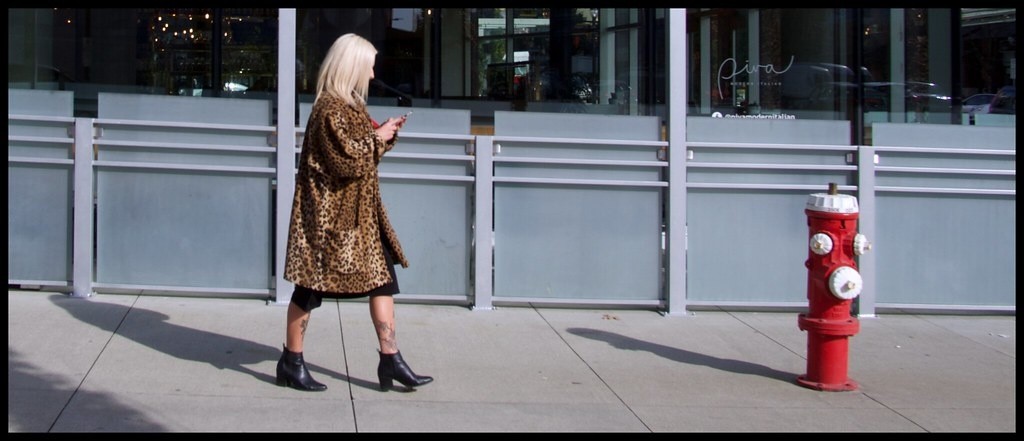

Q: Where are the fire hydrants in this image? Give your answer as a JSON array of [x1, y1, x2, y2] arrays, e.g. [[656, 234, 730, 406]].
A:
[[799, 182, 873, 393]]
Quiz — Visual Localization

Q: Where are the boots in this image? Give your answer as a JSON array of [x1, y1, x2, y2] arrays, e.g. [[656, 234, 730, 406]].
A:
[[276, 343, 327, 391], [377, 348, 433, 389]]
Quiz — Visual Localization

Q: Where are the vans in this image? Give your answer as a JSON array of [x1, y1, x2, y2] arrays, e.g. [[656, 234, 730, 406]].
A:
[[488, 61, 527, 96]]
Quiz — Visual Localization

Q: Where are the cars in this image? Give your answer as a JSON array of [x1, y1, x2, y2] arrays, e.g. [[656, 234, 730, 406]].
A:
[[817, 62, 854, 82], [952, 93, 996, 114], [859, 81, 950, 112], [837, 84, 887, 112], [783, 64, 830, 99]]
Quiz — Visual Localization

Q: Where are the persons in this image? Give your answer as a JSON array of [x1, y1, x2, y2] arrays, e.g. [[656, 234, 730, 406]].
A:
[[276, 33, 434, 391]]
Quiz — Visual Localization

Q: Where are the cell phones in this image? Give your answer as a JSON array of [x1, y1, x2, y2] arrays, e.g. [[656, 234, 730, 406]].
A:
[[401, 111, 412, 119]]
